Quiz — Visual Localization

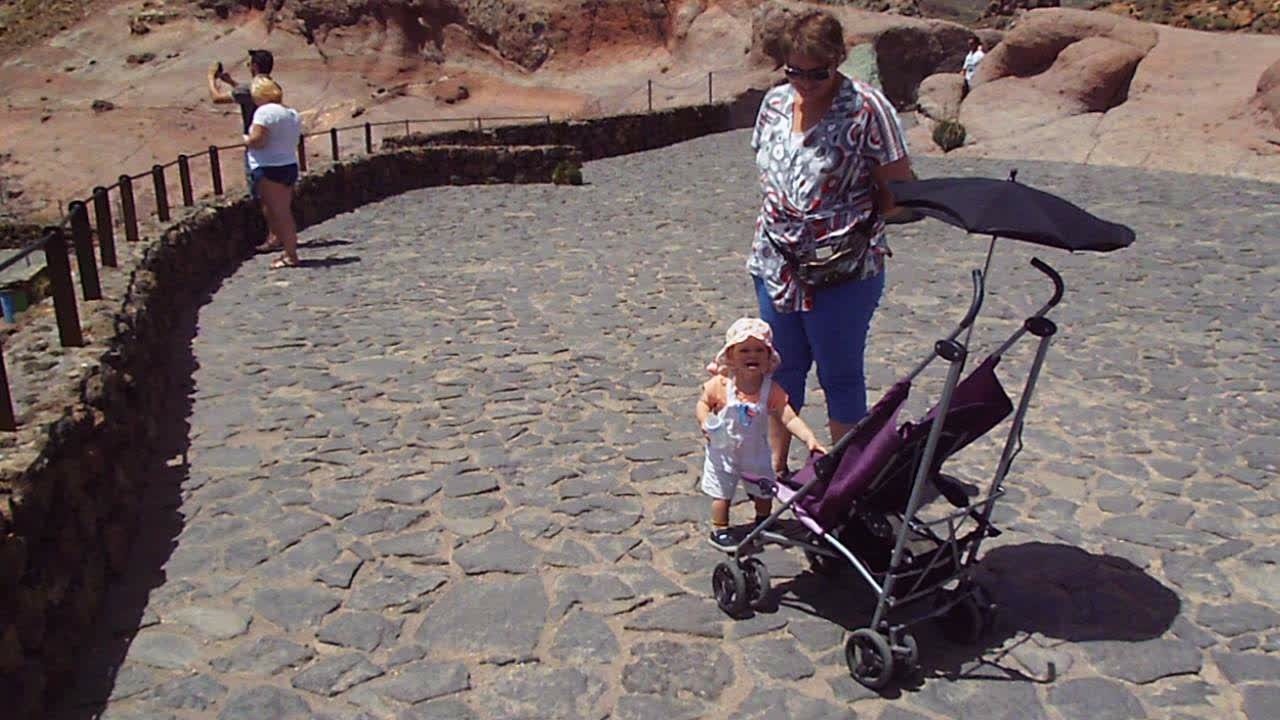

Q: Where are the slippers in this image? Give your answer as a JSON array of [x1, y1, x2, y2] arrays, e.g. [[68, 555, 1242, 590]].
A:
[[269, 257, 299, 269], [272, 254, 289, 261]]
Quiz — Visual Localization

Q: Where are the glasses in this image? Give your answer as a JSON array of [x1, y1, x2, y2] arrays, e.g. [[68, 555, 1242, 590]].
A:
[[783, 62, 833, 81], [245, 62, 256, 67]]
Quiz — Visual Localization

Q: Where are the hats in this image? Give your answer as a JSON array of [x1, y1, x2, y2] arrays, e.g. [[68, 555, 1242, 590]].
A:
[[717, 316, 782, 378]]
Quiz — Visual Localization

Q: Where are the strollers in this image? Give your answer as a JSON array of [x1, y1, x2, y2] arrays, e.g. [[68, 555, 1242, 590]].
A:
[[713, 255, 1064, 692]]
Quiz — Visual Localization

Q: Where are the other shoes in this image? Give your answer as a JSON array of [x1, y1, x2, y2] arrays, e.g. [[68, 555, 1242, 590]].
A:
[[709, 529, 739, 552], [255, 241, 285, 252], [755, 515, 786, 533], [770, 495, 793, 520]]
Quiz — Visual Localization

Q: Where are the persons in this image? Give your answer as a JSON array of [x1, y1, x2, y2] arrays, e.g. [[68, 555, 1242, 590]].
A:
[[240, 76, 301, 269], [695, 317, 829, 551], [207, 49, 274, 242], [744, 13, 913, 478], [960, 35, 985, 99]]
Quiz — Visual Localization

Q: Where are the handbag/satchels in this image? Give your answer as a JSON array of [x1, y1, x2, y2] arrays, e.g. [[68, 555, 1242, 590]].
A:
[[756, 205, 877, 290]]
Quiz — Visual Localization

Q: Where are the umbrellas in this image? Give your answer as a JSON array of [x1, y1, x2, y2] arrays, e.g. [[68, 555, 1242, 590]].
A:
[[888, 168, 1138, 378]]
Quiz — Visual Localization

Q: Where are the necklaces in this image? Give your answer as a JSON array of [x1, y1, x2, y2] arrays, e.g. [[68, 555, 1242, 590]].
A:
[[736, 383, 759, 396]]
